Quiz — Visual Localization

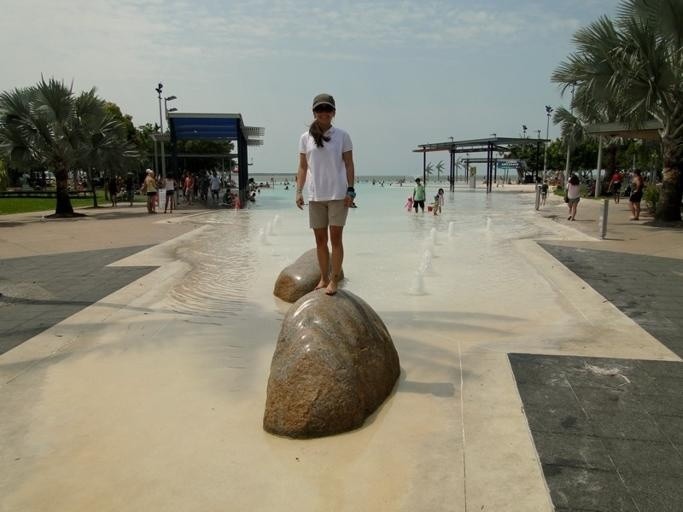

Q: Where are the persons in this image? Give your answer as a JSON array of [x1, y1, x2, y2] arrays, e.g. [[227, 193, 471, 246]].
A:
[[539, 168, 644, 221], [295, 94, 355, 295], [41, 171, 47, 189]]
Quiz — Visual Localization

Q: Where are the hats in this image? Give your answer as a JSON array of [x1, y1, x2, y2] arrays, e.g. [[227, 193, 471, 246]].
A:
[[312, 94, 336, 111]]
